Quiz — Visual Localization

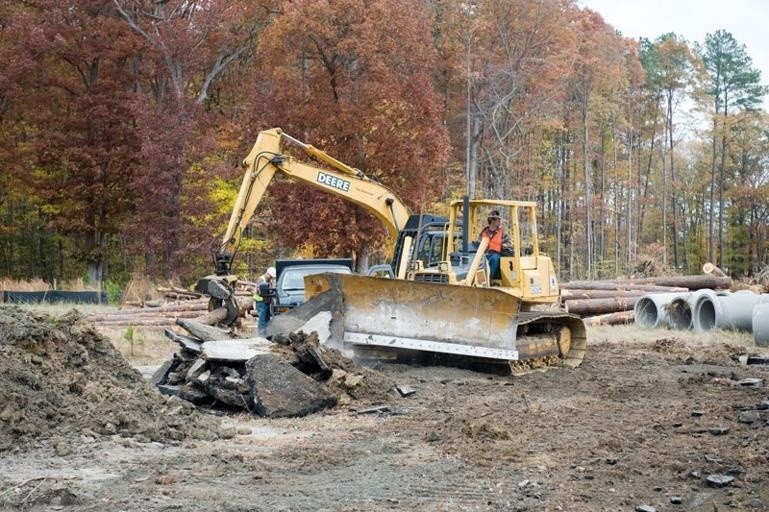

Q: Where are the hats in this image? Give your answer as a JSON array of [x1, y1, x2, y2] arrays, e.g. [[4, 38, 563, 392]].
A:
[[487, 210, 502, 218]]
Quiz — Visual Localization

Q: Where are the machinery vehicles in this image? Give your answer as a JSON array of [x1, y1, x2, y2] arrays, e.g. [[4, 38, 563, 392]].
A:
[[193, 127, 588, 377]]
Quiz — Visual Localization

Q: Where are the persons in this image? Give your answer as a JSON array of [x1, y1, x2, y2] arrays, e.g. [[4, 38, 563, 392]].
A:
[[473, 209, 515, 288], [252, 266, 277, 339]]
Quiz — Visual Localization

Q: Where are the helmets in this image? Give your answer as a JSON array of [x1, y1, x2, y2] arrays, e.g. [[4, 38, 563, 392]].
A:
[[267, 267, 276, 278]]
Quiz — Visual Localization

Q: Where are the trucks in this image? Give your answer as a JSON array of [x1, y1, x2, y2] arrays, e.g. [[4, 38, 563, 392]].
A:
[[259, 258, 397, 320]]
